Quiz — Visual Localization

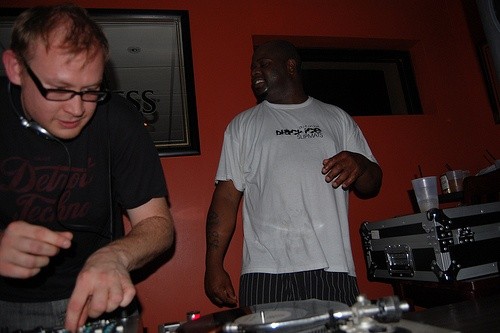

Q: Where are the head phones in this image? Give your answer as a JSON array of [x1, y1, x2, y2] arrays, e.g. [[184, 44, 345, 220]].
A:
[[7, 81, 60, 142]]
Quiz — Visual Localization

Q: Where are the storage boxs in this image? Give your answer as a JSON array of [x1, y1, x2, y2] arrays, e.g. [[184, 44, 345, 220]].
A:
[[360, 203, 500, 282]]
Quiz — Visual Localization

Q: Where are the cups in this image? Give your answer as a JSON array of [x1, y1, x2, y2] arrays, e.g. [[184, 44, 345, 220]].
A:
[[447, 168, 463, 192], [410, 176, 438, 212]]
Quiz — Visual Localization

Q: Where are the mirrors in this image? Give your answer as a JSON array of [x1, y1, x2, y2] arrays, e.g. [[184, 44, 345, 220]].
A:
[[86, 8, 201, 156]]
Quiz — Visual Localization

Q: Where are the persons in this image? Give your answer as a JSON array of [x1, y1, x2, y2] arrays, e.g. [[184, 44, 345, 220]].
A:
[[204, 39, 383, 308], [0, 4, 175, 333]]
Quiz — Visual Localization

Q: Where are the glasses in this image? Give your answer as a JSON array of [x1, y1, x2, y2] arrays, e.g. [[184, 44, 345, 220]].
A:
[[17, 50, 110, 102]]
